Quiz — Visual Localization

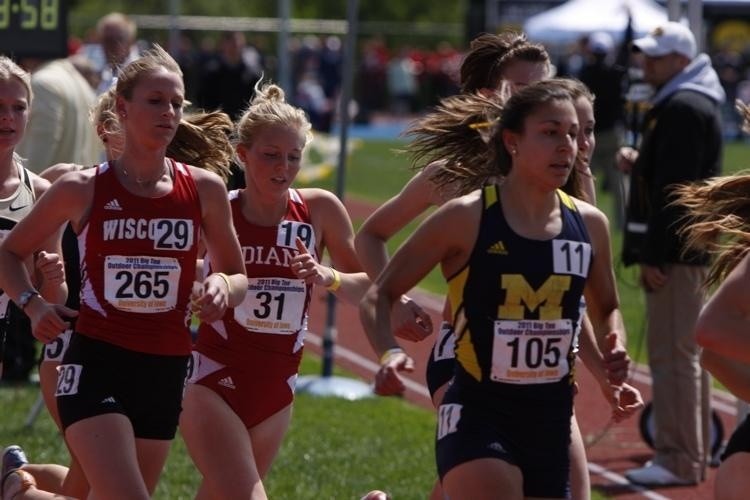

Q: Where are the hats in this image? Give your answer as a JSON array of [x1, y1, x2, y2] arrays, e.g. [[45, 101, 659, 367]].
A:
[[587, 32, 614, 54], [630, 21, 697, 61]]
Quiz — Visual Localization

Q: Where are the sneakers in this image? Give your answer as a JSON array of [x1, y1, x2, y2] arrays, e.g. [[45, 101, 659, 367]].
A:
[[625, 461, 710, 486], [0, 445, 38, 500]]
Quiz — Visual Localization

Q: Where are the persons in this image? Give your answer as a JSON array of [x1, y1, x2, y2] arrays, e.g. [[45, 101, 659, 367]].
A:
[[0, 0, 749, 499]]
